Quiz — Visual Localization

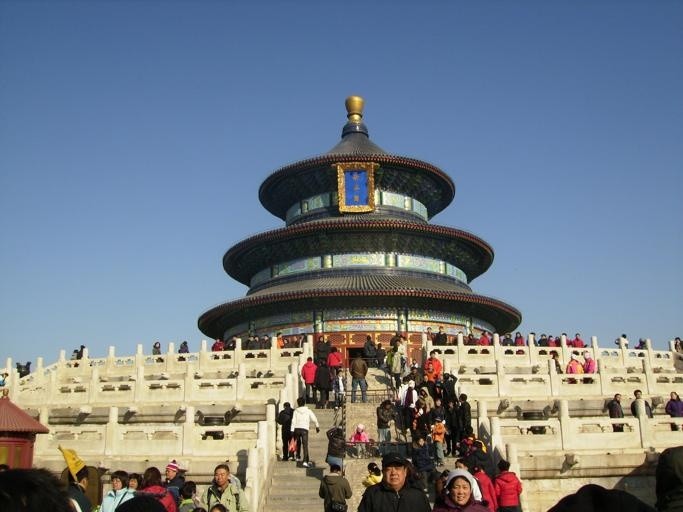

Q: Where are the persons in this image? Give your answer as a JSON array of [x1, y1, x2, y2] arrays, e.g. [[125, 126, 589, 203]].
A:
[[615, 334, 629, 350], [631, 389, 654, 419], [152, 342, 163, 362], [278, 402, 294, 460], [0, 459, 251, 512], [635, 337, 646, 357], [75, 345, 85, 367], [178, 340, 190, 361], [290, 397, 319, 468], [314, 324, 522, 512], [567, 352, 594, 384], [673, 338, 683, 361], [71, 350, 79, 367], [665, 391, 683, 431], [301, 357, 317, 404], [0, 373, 9, 386], [527, 332, 585, 356], [211, 331, 307, 360], [606, 394, 624, 432]]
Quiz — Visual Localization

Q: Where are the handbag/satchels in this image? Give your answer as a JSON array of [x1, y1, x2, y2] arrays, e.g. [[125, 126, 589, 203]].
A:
[[330, 501, 347, 512], [287, 437, 295, 453]]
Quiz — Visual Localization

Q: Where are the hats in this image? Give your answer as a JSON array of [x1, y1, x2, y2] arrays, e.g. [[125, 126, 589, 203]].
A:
[[434, 417, 441, 422], [444, 468, 473, 494], [166, 460, 177, 472], [382, 452, 409, 468]]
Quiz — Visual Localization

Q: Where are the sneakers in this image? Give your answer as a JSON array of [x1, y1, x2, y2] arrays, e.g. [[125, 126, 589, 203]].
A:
[[302, 462, 310, 468]]
